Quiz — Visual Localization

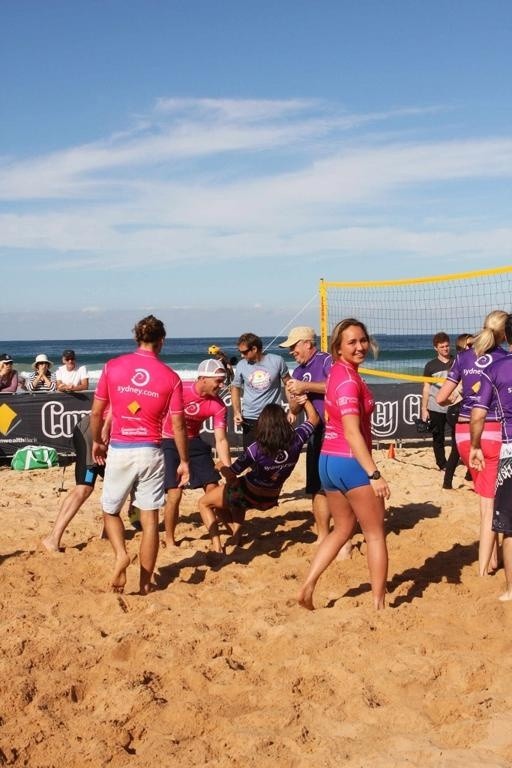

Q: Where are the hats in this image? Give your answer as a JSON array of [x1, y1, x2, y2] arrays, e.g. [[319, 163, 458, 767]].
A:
[[62, 349, 76, 358], [193, 358, 228, 382], [276, 326, 318, 349], [31, 352, 54, 369], [0, 353, 14, 364]]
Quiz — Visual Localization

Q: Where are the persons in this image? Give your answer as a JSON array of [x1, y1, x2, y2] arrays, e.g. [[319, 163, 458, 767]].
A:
[[300, 318, 395, 611], [89, 314, 192, 594], [0, 352, 19, 392], [420, 332, 465, 469], [43, 403, 120, 557], [456, 333, 477, 352], [27, 354, 58, 391], [435, 310, 508, 578], [467, 313, 512, 600], [208, 343, 235, 382], [54, 350, 89, 392]]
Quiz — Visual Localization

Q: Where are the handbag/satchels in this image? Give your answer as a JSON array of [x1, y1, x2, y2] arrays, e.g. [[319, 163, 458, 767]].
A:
[[10, 443, 61, 471]]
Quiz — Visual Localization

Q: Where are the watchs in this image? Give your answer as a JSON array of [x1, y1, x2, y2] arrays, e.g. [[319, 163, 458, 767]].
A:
[[366, 471, 382, 480]]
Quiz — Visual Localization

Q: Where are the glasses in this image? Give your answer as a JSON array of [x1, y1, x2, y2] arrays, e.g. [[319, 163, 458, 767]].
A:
[[238, 347, 255, 355]]
